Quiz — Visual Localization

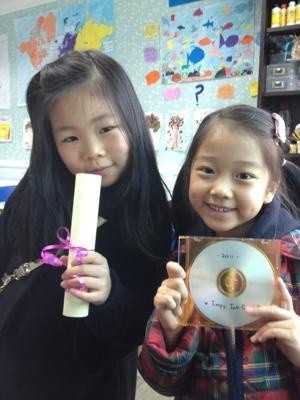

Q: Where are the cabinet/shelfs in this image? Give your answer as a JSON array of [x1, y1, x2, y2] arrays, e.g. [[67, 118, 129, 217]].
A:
[[256, 0, 300, 167]]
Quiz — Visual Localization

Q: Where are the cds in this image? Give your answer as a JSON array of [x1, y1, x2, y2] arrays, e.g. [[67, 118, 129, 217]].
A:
[[189, 240, 276, 327]]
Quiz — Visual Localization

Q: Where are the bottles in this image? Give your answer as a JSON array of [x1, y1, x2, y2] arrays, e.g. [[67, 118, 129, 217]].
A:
[[271, 1, 300, 28]]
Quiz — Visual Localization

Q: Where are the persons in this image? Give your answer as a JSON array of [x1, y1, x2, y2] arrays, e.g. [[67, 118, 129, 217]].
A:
[[136, 103, 299, 399], [0, 50, 175, 399]]
